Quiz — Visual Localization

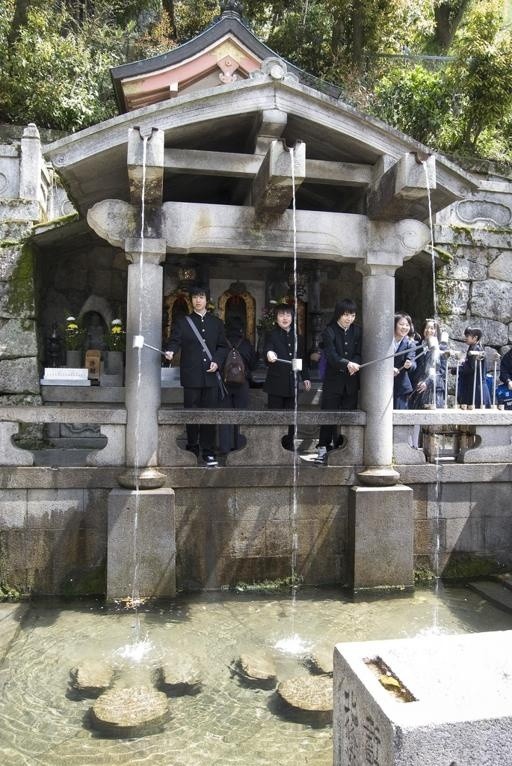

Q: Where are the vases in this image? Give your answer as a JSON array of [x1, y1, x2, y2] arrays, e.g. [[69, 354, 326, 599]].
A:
[[106, 352, 123, 373], [66, 348, 82, 369]]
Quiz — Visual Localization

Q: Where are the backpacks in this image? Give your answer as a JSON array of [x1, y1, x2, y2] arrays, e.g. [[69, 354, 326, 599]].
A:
[[221, 335, 249, 387]]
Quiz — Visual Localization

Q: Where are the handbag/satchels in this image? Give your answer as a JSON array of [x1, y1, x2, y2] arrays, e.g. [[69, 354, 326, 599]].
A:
[[496, 381, 512, 409]]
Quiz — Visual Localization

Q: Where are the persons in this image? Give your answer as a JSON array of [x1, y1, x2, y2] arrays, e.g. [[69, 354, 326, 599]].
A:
[[409, 318, 447, 409], [314, 297, 363, 465], [410, 322, 422, 343], [310, 343, 327, 382], [262, 303, 312, 409], [451, 326, 491, 409], [394, 310, 417, 409], [165, 285, 225, 466], [215, 315, 259, 454], [499, 347, 512, 391]]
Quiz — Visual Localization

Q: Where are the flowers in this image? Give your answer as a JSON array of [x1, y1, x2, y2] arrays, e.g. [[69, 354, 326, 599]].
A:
[[100, 319, 126, 353], [58, 313, 87, 348]]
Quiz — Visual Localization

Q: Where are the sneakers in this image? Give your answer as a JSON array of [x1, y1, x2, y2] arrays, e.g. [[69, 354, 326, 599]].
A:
[[202, 455, 219, 467], [313, 446, 329, 465]]
